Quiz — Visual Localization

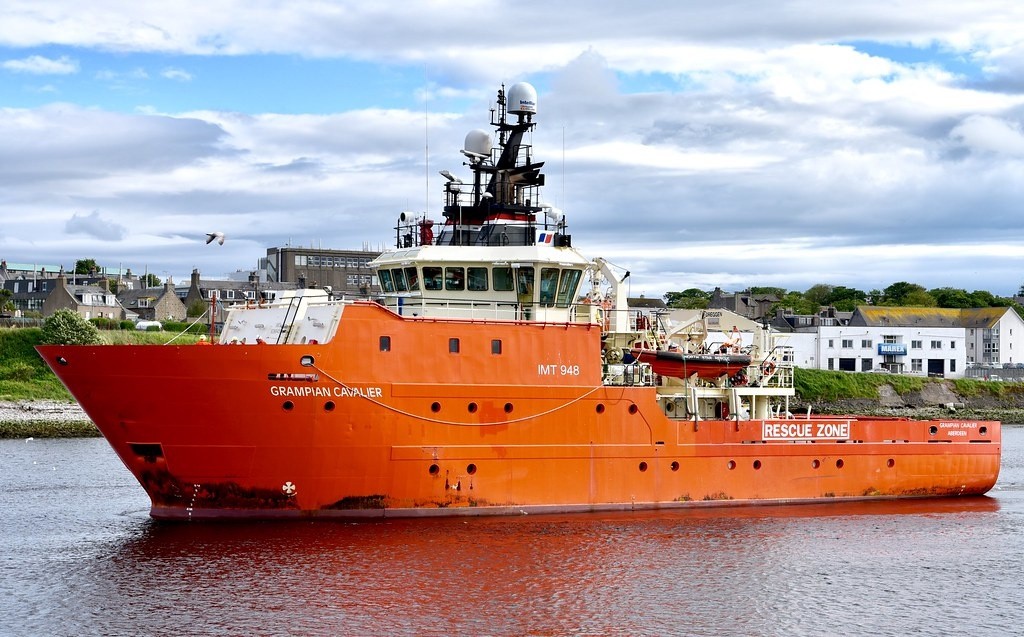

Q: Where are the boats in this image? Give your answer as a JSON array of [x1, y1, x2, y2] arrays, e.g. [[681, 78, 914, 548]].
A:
[[32, 79, 1003, 520]]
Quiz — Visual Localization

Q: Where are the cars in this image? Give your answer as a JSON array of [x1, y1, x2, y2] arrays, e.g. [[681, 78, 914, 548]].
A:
[[1018, 377, 1024, 382], [990, 374, 1004, 381], [0, 312, 11, 318], [865, 368, 891, 374], [966, 362, 1024, 369], [1005, 377, 1016, 382]]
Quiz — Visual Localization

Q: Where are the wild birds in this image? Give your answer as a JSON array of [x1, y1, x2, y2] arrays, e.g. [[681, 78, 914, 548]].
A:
[[206, 232, 225, 245]]
